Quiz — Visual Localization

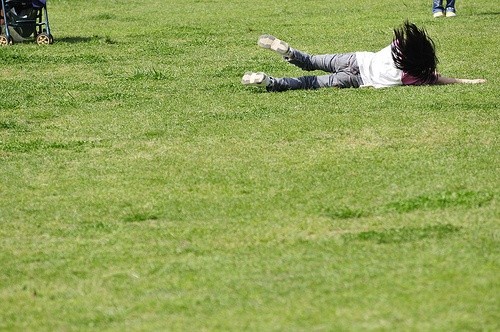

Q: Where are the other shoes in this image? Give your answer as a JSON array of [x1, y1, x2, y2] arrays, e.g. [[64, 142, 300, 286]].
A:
[[434, 12, 445, 18], [446, 11, 456, 17]]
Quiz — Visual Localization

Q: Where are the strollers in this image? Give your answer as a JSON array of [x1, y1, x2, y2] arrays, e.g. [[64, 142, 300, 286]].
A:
[[0, 0, 55, 48]]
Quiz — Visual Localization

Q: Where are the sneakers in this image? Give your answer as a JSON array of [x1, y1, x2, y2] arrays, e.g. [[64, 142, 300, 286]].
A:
[[241, 72, 270, 86], [258, 35, 294, 58]]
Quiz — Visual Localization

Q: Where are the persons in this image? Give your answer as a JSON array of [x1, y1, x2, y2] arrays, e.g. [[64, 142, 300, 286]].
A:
[[241, 20, 487, 92], [432, 0, 457, 18]]
